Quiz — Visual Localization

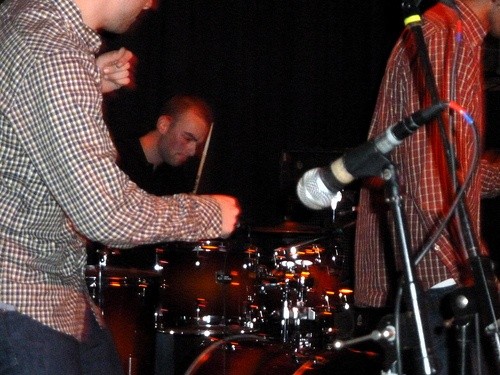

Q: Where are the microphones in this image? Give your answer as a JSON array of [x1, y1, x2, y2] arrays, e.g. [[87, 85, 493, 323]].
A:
[[296, 102, 446, 210]]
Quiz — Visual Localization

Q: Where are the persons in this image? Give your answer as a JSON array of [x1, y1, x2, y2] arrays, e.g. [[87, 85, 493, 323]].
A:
[[0, 0, 242, 375], [353, 1, 500, 375], [86, 97, 214, 270]]
